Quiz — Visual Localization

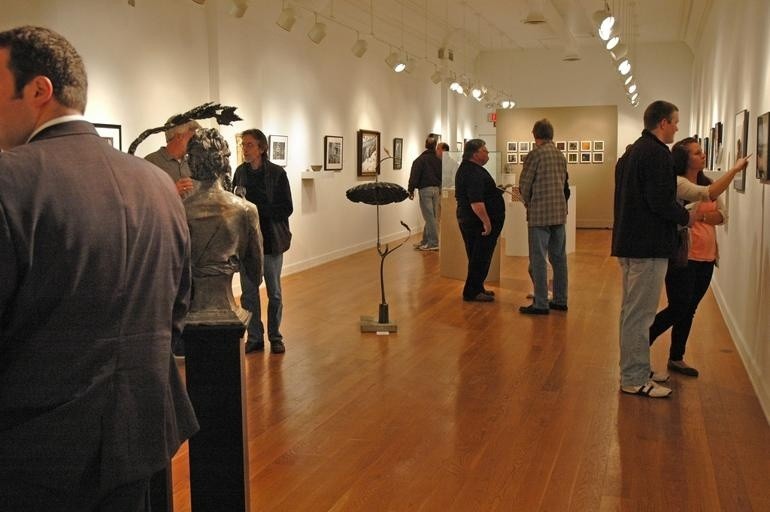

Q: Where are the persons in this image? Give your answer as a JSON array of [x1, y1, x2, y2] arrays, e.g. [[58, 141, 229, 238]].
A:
[[233, 129, 294, 354], [408, 137, 442, 251], [648, 138, 753, 376], [519, 119, 568, 314], [455, 139, 505, 301], [157, 128, 264, 325], [145, 116, 204, 201], [436, 143, 459, 235], [527, 171, 570, 301], [611, 101, 699, 398], [0, 26, 192, 512]]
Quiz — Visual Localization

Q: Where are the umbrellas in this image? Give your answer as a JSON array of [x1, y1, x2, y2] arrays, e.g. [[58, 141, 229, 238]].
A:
[[346, 172, 409, 256]]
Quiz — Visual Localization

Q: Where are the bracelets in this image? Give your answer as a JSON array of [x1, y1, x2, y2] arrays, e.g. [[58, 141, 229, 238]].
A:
[[702, 213, 707, 223]]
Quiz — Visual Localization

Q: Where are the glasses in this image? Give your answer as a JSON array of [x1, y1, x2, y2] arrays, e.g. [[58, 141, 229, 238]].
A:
[[239, 142, 262, 148]]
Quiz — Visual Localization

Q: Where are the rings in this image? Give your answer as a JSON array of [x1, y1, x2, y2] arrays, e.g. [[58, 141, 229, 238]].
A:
[[183, 186, 187, 190]]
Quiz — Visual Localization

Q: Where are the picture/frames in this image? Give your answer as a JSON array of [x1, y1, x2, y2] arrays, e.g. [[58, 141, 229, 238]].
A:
[[506, 141, 605, 164], [324, 136, 343, 170], [393, 138, 402, 170], [357, 129, 380, 177], [93, 123, 122, 151], [693, 110, 770, 193], [269, 135, 288, 167]]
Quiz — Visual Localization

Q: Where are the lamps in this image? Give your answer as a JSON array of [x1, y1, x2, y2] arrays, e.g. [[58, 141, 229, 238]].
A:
[[191, 0, 249, 18], [590, 0, 641, 109], [425, 0, 516, 109], [275, 0, 420, 74]]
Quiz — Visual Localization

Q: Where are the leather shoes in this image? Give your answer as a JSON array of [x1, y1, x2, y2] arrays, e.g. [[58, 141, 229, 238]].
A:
[[461, 290, 496, 303]]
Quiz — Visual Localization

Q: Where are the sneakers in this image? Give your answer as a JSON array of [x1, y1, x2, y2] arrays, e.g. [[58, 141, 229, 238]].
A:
[[517, 305, 551, 316], [620, 378, 674, 399], [411, 240, 440, 252], [269, 340, 286, 355], [666, 358, 700, 378], [548, 298, 569, 312], [244, 340, 265, 354], [648, 369, 672, 383]]
